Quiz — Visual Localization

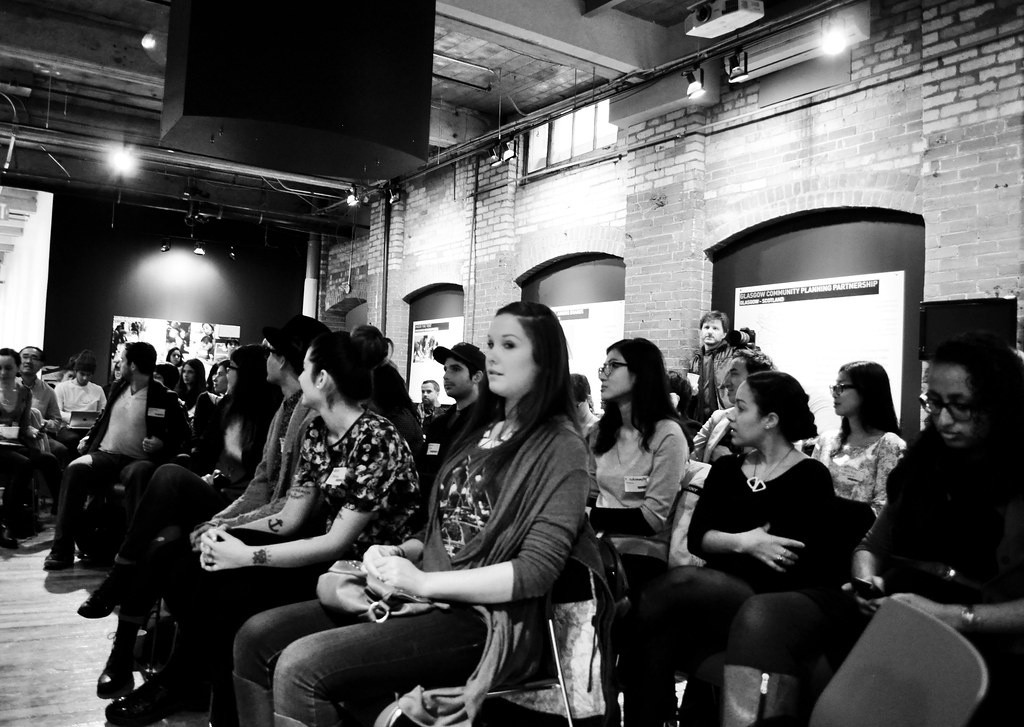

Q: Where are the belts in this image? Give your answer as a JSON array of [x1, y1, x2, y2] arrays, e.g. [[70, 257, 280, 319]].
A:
[[902, 559, 970, 586]]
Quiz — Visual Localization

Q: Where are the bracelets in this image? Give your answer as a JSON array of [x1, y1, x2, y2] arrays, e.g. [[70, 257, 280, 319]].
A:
[[396, 545, 406, 557], [961, 604, 977, 629]]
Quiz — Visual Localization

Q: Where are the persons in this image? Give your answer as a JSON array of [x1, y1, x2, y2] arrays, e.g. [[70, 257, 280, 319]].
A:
[[198, 336, 215, 361], [201, 323, 217, 339], [225, 339, 239, 358], [0, 301, 1024, 727]]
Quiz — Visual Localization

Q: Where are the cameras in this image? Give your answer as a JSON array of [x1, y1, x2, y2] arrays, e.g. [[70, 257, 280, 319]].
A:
[[727, 329, 756, 350]]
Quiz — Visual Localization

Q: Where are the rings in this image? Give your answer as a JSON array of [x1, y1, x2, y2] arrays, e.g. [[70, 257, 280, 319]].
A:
[[777, 556, 783, 561]]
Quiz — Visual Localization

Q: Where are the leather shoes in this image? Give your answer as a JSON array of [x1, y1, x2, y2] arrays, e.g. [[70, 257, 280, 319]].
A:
[[0, 519, 18, 547], [104, 669, 211, 727]]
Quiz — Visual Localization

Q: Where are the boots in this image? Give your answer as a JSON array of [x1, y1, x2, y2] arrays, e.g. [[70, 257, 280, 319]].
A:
[[77, 553, 147, 619], [718, 665, 798, 727], [231, 671, 275, 727], [97, 614, 148, 699]]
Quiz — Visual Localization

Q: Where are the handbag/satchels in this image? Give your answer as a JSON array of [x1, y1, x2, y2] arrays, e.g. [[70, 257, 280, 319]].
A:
[[494, 567, 609, 719], [315, 559, 452, 624]]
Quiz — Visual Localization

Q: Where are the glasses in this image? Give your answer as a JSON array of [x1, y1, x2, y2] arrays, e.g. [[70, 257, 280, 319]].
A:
[[262, 344, 281, 357], [598, 361, 629, 375], [225, 364, 239, 373], [23, 355, 43, 362], [831, 384, 856, 394], [916, 389, 978, 423]]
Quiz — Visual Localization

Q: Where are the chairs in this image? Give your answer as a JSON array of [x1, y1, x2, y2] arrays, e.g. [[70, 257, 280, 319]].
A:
[[477, 457, 990, 727]]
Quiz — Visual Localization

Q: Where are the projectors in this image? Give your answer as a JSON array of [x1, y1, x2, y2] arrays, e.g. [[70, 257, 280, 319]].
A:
[[685, 0, 764, 39]]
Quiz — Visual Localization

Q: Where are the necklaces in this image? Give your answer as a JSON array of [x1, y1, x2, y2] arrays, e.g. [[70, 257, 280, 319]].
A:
[[746, 446, 795, 493]]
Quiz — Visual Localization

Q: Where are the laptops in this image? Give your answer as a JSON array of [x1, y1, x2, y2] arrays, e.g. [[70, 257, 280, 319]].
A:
[[66, 411, 101, 429]]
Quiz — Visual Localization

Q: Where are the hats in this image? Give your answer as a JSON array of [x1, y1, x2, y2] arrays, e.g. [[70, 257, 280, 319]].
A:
[[73, 349, 97, 376], [151, 364, 180, 385], [260, 312, 333, 368], [431, 342, 487, 372]]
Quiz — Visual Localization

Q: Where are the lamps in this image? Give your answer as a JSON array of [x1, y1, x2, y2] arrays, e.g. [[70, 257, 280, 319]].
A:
[[489, 141, 517, 168], [681, 62, 702, 97], [162, 184, 401, 255], [727, 48, 749, 84]]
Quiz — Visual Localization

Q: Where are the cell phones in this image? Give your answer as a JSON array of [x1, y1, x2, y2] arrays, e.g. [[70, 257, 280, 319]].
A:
[[853, 577, 883, 601]]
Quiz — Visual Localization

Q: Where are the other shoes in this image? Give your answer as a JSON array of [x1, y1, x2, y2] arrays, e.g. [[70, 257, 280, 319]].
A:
[[44, 550, 73, 570]]
